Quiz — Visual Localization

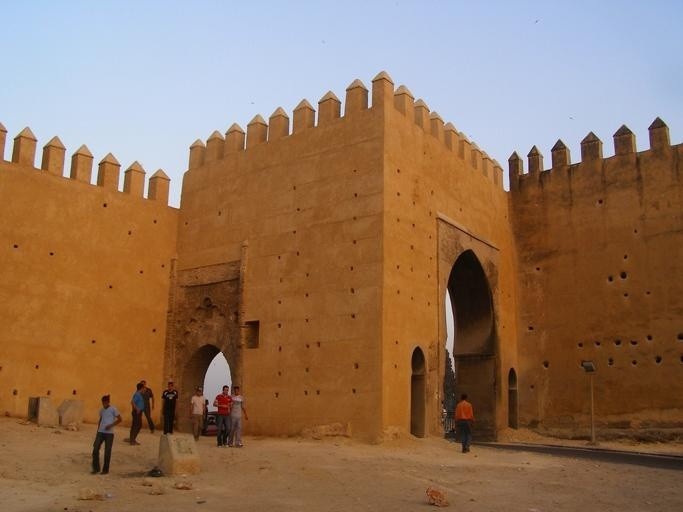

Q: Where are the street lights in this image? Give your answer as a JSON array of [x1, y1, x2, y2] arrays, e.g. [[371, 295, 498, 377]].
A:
[[581, 360, 598, 444]]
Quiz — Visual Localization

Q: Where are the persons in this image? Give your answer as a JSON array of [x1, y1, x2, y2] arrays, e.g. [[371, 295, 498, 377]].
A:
[[139, 380, 156, 433], [204, 399, 209, 435], [128, 382, 145, 445], [188, 387, 206, 441], [160, 381, 178, 436], [454, 394, 477, 453], [228, 385, 248, 447], [90, 393, 122, 475], [212, 385, 234, 447]]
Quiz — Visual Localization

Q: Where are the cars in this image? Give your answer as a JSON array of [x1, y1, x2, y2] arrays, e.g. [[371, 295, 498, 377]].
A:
[[204, 410, 225, 437]]
